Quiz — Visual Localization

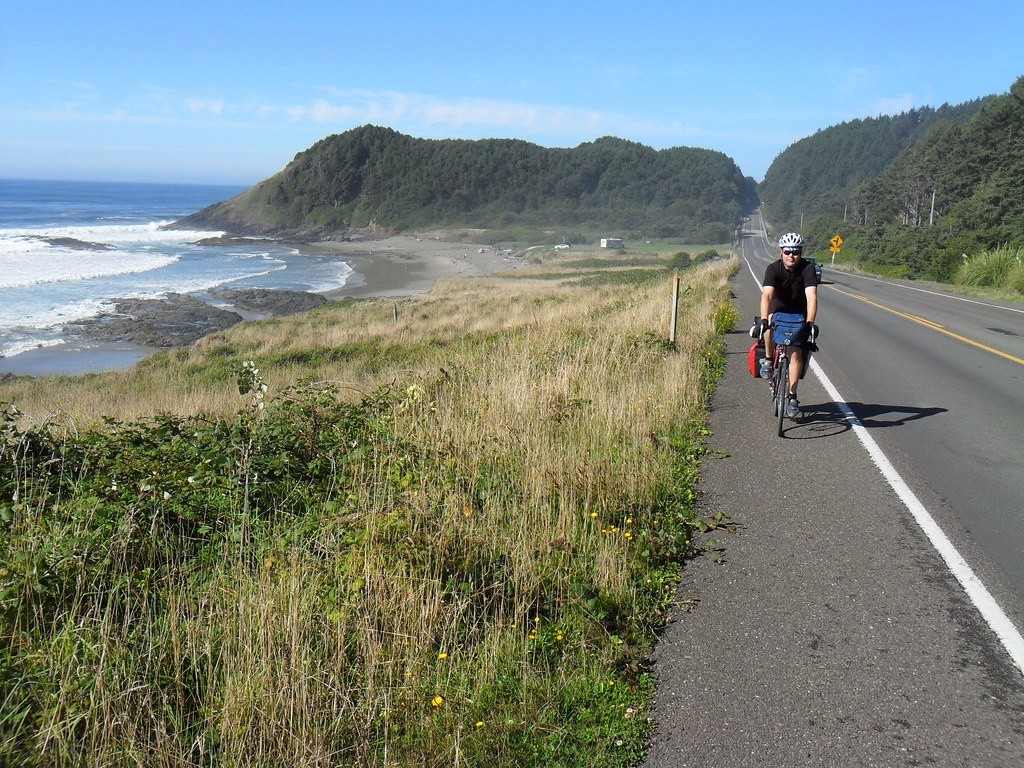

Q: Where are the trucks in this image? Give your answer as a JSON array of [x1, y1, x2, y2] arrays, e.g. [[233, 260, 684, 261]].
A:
[[600, 237, 625, 249]]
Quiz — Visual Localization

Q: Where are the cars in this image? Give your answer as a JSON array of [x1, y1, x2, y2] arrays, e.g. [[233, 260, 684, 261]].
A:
[[555, 243, 570, 250], [802, 256, 823, 284]]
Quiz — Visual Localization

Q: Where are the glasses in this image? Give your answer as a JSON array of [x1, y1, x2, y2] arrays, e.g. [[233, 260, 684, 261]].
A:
[[783, 250, 801, 256]]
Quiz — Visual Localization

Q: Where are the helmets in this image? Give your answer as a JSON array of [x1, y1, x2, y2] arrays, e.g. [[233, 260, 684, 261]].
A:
[[777, 233, 804, 250]]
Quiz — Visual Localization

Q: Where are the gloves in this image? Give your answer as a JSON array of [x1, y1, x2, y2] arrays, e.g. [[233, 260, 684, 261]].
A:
[[760, 318, 768, 333], [811, 323, 820, 338]]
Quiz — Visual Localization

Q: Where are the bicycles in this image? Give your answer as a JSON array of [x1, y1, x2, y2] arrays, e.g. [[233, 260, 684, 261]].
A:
[[757, 321, 815, 436]]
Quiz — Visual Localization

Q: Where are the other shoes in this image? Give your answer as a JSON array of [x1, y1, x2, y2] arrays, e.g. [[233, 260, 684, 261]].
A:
[[787, 398, 799, 419], [760, 361, 773, 379]]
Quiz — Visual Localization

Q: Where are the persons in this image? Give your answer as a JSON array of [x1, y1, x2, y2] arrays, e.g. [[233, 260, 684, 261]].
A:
[[758, 233, 819, 417]]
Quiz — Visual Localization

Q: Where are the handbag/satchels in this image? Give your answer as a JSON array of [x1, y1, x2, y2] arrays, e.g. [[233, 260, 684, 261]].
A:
[[773, 312, 805, 346]]
